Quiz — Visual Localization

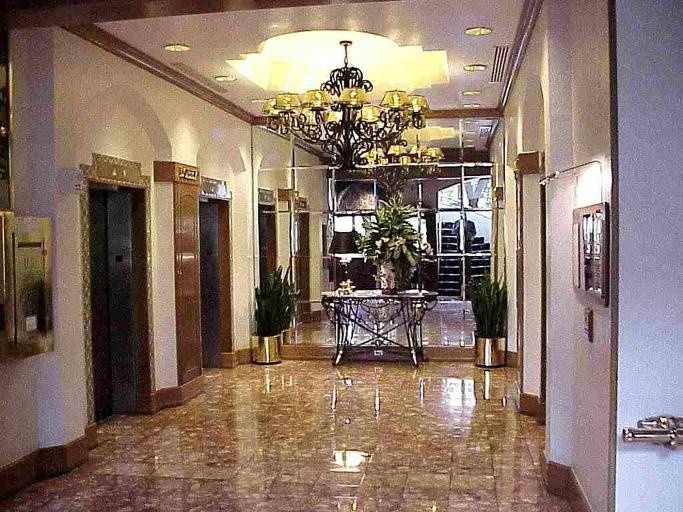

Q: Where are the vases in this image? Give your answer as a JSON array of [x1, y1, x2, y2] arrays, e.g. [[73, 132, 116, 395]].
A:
[[374, 257, 398, 295]]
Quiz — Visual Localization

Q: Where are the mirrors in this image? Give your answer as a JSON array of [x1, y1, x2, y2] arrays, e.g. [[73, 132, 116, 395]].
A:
[[248, 115, 506, 360]]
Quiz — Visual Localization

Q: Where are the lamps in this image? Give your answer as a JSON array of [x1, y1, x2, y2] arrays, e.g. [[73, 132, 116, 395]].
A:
[[355, 127, 446, 181], [263, 40, 428, 172]]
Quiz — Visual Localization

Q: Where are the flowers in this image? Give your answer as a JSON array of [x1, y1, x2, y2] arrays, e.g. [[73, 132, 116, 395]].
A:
[[355, 190, 435, 287]]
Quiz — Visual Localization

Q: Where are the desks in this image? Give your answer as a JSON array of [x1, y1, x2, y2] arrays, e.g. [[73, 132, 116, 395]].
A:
[[318, 287, 441, 367]]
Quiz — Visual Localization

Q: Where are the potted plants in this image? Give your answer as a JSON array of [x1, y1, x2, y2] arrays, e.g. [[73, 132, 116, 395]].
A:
[[251, 266, 294, 365], [468, 274, 509, 368]]
